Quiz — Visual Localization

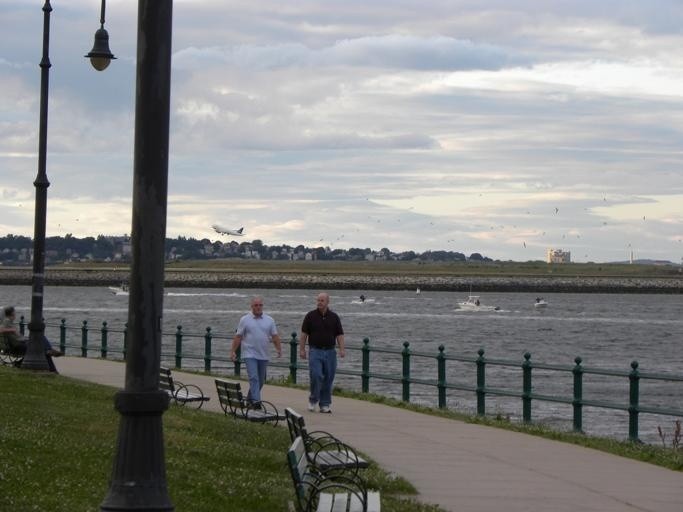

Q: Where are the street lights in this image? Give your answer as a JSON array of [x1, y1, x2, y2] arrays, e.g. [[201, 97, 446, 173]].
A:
[[21, 0, 117, 374]]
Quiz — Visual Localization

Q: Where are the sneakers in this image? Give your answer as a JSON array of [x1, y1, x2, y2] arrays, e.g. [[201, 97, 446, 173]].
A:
[[308, 400, 315, 412], [320, 408, 331, 412]]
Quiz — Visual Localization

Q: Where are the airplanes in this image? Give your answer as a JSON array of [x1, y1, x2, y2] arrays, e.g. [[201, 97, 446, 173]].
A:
[[209, 223, 246, 236]]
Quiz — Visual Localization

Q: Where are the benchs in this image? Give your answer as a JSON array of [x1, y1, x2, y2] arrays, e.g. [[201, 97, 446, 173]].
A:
[[159, 366, 210, 409], [215, 379, 286, 428], [286, 436, 381, 512], [0, 333, 25, 367], [284, 406, 369, 486]]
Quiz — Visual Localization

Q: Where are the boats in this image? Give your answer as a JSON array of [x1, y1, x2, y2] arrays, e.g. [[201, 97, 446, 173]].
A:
[[535, 297, 548, 311], [107, 283, 129, 294], [457, 296, 500, 312], [351, 297, 374, 304]]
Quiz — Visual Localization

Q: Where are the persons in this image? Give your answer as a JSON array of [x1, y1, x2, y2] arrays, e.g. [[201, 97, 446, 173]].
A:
[[0, 305, 65, 373], [299, 291, 346, 413], [230, 297, 283, 407]]
